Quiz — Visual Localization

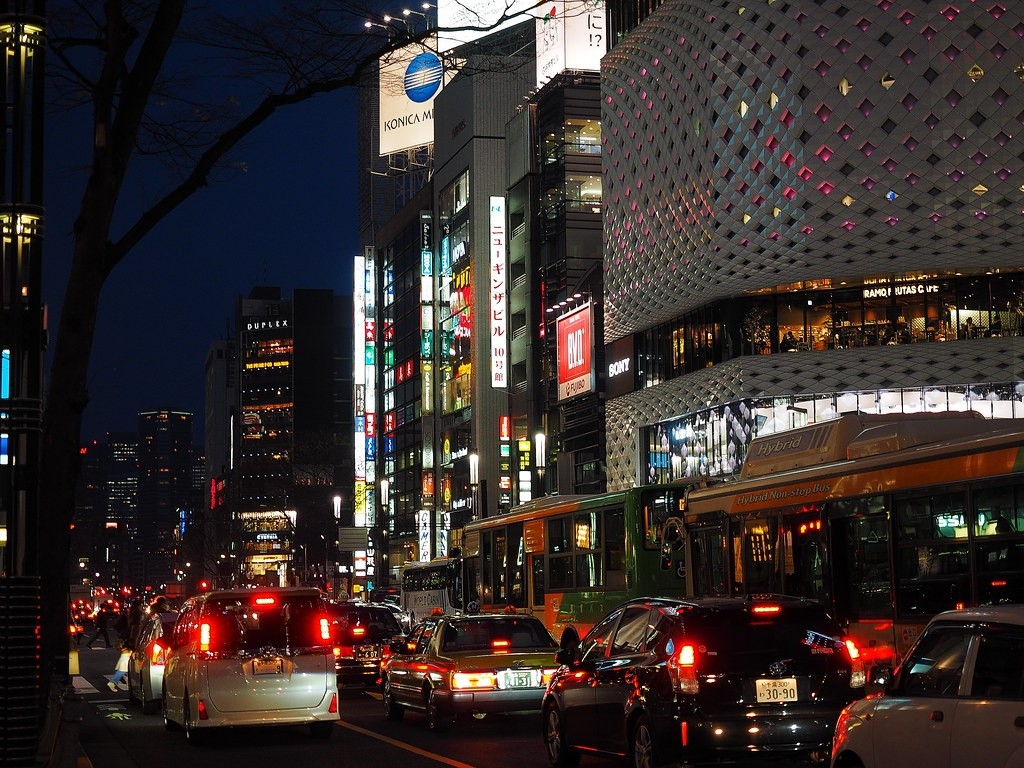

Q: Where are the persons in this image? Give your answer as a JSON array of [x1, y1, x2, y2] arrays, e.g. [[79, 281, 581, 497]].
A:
[[958, 316, 1001, 340], [431, 607, 445, 617], [68, 603, 114, 649], [779, 332, 804, 352], [107, 597, 172, 692], [504, 605, 517, 615], [828, 324, 912, 349], [758, 336, 766, 352], [466, 602, 480, 616]]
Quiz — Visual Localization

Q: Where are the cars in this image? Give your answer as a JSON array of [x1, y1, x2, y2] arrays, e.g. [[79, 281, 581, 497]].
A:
[[328, 602, 408, 692], [116, 581, 416, 706], [828, 602, 1024, 768], [377, 605, 563, 735], [539, 596, 867, 768]]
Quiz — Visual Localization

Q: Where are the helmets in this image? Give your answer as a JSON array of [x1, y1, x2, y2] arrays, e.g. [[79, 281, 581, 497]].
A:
[[467, 601, 479, 612], [505, 605, 516, 613]]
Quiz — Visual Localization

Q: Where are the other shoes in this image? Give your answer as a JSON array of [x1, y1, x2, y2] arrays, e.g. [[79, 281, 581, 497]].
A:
[[85, 643, 91, 649], [105, 644, 114, 648], [107, 682, 117, 691]]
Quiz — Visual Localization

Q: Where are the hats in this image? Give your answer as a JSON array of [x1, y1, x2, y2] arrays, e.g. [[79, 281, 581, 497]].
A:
[[99, 603, 107, 608]]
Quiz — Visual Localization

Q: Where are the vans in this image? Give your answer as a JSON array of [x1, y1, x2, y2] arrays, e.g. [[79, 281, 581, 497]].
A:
[[155, 589, 341, 740]]
